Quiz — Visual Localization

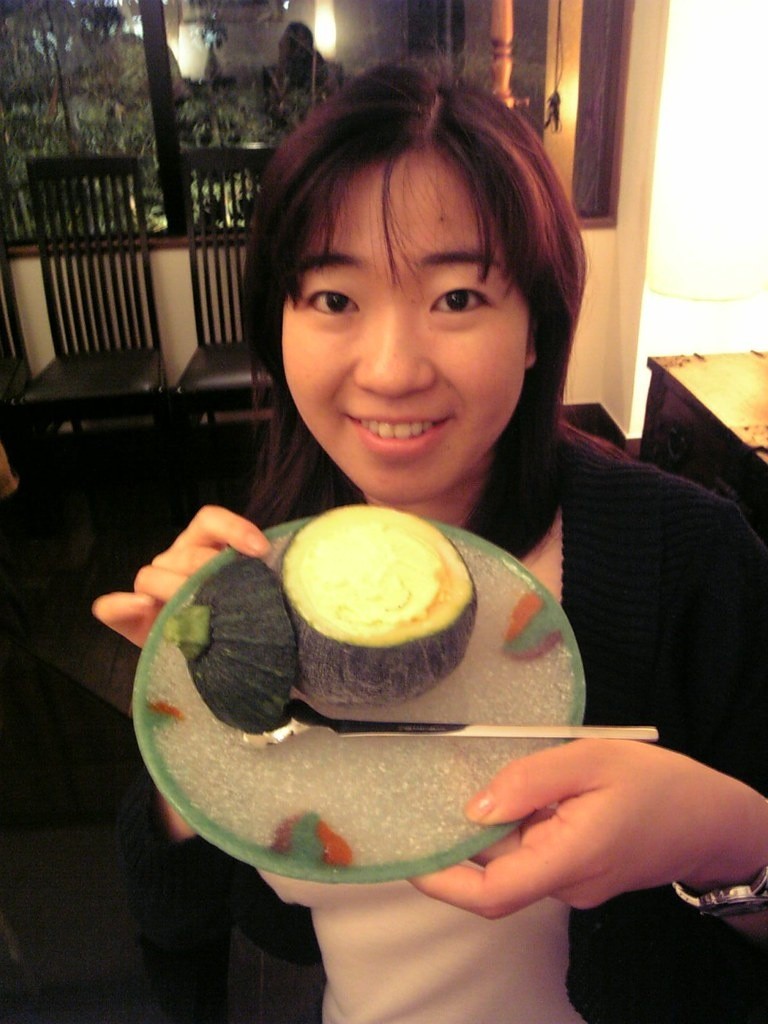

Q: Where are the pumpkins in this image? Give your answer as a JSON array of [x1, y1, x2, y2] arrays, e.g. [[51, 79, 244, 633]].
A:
[[162, 499, 478, 731]]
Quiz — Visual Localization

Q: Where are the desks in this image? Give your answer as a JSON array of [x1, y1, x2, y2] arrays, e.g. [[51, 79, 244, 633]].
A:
[[639, 348, 766, 540]]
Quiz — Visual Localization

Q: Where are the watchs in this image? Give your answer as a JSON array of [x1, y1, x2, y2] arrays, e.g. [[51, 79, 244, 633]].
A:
[[672, 867, 768, 915]]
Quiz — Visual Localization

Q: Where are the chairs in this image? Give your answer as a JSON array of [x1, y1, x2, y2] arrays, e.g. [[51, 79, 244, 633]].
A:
[[171, 144, 276, 523], [10, 154, 168, 526], [0, 239, 32, 468]]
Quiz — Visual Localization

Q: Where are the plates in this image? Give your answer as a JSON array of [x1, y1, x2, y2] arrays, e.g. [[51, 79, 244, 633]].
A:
[[133, 515, 587, 885]]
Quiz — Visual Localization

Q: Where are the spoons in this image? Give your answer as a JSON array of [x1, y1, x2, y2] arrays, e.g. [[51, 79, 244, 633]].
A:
[[242, 698, 659, 749]]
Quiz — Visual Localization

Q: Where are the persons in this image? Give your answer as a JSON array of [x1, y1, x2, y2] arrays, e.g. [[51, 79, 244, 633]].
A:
[[278, 22, 324, 69], [91, 62, 768, 1023]]
